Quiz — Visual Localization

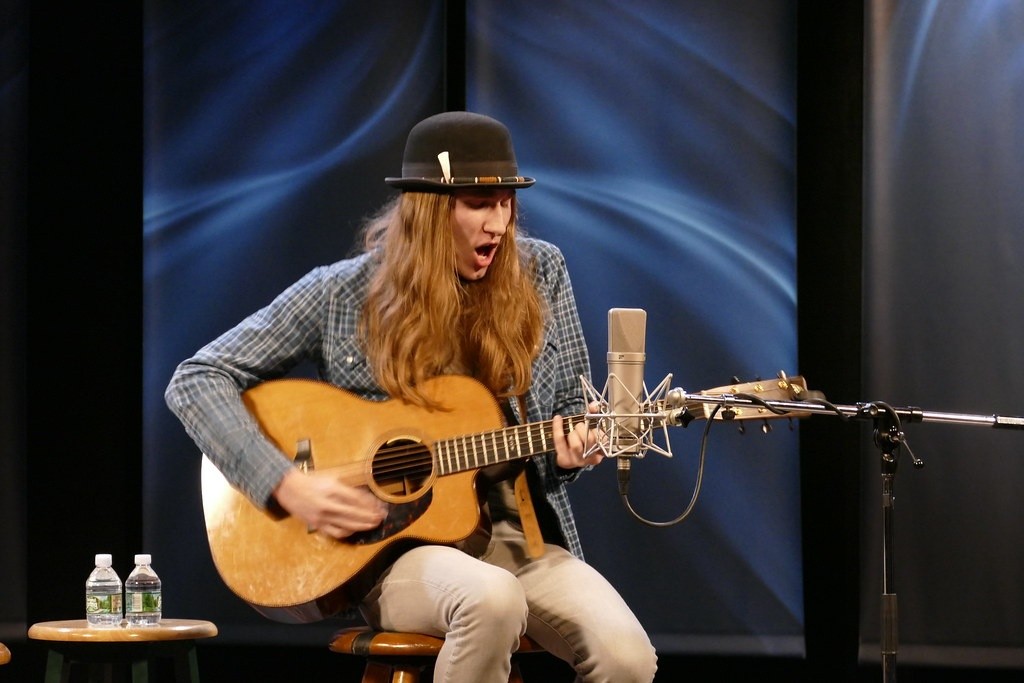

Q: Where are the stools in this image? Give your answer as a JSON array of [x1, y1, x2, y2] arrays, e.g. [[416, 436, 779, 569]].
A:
[[326, 626, 543, 683], [27, 618, 220, 683]]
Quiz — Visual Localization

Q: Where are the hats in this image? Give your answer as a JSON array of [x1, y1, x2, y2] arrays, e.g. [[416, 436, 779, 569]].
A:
[[383, 111, 535, 189]]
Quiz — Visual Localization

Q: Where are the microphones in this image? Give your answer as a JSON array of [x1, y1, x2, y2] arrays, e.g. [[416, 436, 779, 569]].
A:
[[607, 307, 646, 496]]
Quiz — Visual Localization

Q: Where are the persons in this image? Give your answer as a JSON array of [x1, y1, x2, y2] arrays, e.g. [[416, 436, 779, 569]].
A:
[[165, 112, 657, 683]]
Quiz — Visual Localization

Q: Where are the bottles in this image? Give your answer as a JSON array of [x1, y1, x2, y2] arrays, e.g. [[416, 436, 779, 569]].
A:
[[84, 555, 122, 629], [126, 553, 161, 626]]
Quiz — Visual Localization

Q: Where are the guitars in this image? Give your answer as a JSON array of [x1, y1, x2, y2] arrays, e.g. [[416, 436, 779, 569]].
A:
[[199, 368, 827, 625]]
